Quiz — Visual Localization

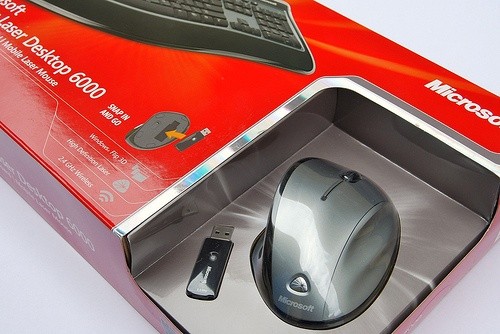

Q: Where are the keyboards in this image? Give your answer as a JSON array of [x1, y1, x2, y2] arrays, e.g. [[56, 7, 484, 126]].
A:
[[29, 0, 316, 75]]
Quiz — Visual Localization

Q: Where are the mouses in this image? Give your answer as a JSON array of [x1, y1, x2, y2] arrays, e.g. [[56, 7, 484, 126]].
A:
[[249, 157, 400, 330], [124, 112, 190, 150]]
